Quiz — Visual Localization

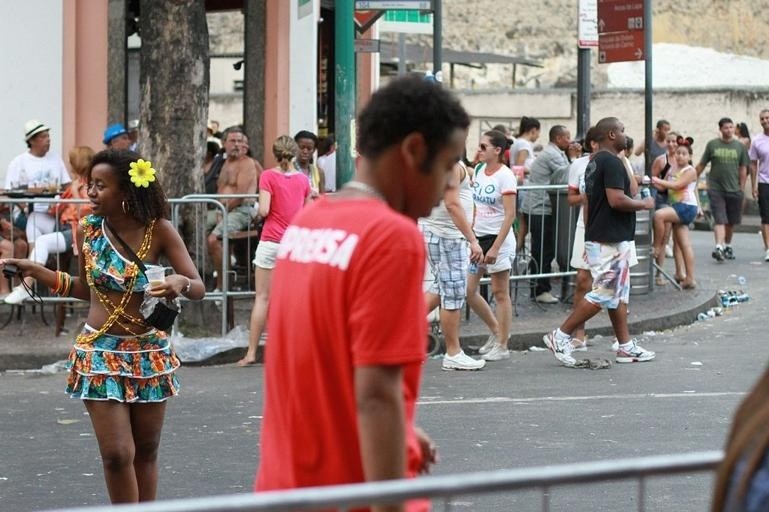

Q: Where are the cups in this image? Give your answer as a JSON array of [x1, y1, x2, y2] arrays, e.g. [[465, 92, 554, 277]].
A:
[[7, 173, 62, 197], [143, 267, 166, 292]]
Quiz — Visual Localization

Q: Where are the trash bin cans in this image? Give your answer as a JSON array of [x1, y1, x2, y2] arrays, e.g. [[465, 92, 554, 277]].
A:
[[629, 208, 651, 294]]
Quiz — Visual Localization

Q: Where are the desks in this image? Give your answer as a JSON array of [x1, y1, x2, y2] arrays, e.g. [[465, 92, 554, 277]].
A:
[[0, 191, 64, 330]]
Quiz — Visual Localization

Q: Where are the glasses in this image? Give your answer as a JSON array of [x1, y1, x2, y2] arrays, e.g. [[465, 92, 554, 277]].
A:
[[478, 142, 499, 152]]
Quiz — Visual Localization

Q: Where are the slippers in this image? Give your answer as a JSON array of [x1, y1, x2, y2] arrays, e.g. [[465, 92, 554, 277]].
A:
[[236, 357, 258, 367]]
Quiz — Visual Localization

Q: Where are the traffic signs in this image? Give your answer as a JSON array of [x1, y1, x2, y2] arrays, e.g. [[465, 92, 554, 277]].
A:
[[571, 0, 651, 65]]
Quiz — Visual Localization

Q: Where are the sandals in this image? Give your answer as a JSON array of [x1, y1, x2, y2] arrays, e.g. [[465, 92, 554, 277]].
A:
[[571, 335, 588, 352]]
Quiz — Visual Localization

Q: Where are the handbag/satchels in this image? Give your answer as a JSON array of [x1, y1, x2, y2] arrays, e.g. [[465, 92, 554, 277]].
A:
[[138, 281, 184, 333]]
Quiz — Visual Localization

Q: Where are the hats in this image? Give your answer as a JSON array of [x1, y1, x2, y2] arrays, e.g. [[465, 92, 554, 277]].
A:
[[23, 119, 52, 142], [102, 122, 130, 145]]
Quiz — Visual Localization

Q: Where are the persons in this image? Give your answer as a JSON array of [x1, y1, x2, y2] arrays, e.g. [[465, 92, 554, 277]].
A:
[[252, 74, 469, 512], [635, 109, 769, 289], [417, 118, 656, 369], [204, 121, 336, 364], [0, 147, 206, 506], [709, 369, 769, 512], [0, 119, 139, 303]]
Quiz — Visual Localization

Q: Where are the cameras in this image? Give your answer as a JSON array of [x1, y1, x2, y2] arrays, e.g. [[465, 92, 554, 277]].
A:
[[2, 264, 18, 279]]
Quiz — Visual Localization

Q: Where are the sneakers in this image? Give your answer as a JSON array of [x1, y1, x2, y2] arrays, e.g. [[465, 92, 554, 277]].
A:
[[711, 247, 726, 264], [3, 285, 36, 306], [763, 248, 769, 261], [540, 326, 579, 368], [478, 330, 513, 355], [724, 245, 737, 261], [440, 349, 487, 371], [650, 245, 699, 290], [211, 286, 224, 308], [530, 292, 559, 303], [615, 342, 657, 364], [480, 344, 512, 361]]
